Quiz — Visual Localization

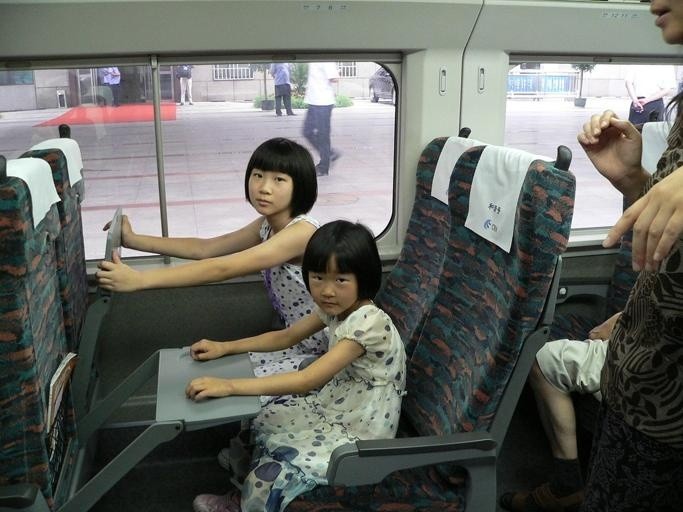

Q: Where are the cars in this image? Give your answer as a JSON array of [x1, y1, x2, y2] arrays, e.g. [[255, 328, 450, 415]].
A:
[[366, 65, 396, 106]]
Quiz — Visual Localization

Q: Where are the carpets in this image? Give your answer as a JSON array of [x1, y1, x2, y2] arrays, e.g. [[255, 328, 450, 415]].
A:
[[31, 104, 176, 128]]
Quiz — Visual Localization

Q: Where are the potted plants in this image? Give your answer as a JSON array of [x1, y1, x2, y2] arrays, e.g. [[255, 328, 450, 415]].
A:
[[249, 64, 274, 110]]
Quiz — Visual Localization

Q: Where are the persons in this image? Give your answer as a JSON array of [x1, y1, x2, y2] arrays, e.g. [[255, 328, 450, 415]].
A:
[[178, 214, 427, 512], [623, 63, 678, 133], [579, 0, 681, 509], [499, 308, 625, 511], [267, 63, 296, 117], [96, 67, 122, 108], [301, 62, 344, 178], [94, 135, 324, 471], [175, 62, 196, 105]]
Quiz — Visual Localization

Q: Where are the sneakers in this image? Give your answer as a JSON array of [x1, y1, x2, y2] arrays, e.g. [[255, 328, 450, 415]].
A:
[[316, 152, 337, 176], [218, 448, 232, 470], [193, 487, 240, 512], [501, 482, 587, 511], [276, 112, 296, 116]]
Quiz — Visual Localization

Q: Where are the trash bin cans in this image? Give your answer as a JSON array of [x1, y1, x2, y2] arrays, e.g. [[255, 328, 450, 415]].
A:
[[57, 89, 67, 109]]
